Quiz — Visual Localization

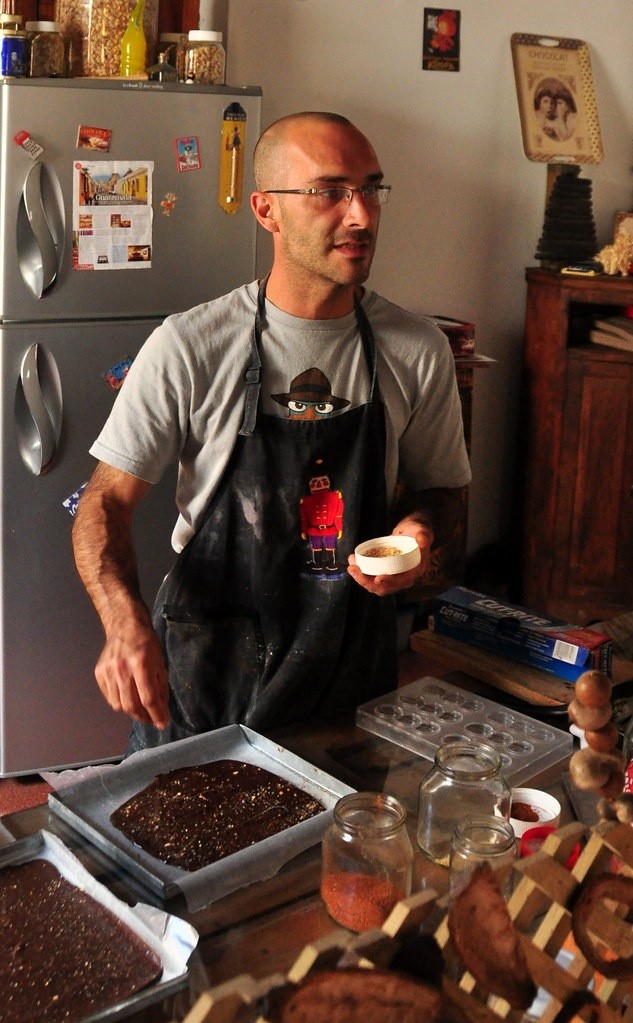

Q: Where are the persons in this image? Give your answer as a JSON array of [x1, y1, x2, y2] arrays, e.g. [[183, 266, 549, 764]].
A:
[[69, 111, 471, 751], [534, 83, 576, 139]]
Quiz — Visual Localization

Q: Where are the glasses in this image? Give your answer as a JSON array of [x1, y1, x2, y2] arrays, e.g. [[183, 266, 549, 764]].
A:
[[263, 185, 391, 210]]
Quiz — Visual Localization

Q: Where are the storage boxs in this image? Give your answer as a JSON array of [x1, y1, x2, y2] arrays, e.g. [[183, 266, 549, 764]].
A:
[[429, 584, 616, 682]]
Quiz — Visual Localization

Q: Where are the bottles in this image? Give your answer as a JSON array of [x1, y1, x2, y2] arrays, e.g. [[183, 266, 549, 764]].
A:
[[320, 792, 415, 933], [24, 21, 65, 79], [416, 742, 513, 867], [448, 812, 518, 906], [153, 32, 187, 80], [511, 826, 581, 915], [0, 13, 29, 78], [185, 30, 225, 85]]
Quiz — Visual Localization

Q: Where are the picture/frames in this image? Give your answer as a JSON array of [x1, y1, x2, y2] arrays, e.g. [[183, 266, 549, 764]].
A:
[[510, 32, 605, 164]]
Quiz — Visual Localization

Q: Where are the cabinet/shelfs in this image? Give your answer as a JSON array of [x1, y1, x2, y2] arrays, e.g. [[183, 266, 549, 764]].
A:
[[520, 266, 633, 631]]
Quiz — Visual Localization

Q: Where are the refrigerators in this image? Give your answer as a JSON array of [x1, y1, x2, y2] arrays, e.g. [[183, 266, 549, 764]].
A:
[[2, 79, 262, 780]]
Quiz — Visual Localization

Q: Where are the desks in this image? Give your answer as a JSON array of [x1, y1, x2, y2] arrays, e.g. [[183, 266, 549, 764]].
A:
[[0, 609, 633, 1023]]
